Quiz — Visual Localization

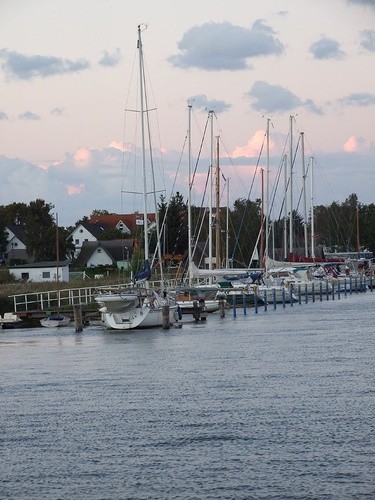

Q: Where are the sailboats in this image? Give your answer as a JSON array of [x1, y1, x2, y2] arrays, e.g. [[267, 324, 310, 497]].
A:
[[93, 21, 375, 331]]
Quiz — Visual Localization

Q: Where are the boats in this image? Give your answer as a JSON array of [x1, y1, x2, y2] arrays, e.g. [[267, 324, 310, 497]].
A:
[[40, 314, 71, 328]]
